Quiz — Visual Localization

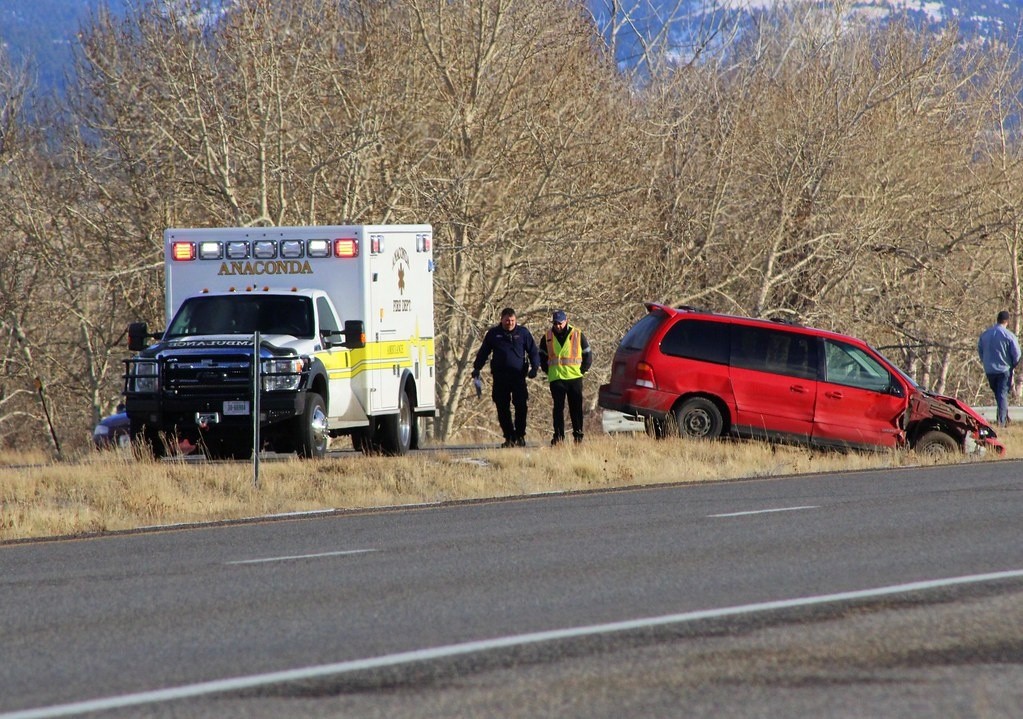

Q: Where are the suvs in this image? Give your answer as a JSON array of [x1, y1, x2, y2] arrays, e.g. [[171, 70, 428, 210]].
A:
[[596, 301, 1007, 464]]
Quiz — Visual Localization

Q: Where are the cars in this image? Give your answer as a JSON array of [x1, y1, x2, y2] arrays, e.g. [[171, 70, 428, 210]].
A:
[[91, 408, 203, 456]]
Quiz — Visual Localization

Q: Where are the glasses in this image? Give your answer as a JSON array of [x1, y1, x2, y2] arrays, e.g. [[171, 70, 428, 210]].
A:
[[553, 320, 564, 325]]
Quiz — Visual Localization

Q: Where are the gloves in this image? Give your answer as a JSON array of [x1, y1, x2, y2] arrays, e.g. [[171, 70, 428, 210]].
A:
[[472, 368, 480, 378], [528, 367, 538, 378]]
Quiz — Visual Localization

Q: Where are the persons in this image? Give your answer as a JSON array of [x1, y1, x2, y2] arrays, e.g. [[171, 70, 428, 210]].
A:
[[471, 307, 539, 447], [975, 311, 1022, 427], [540, 311, 593, 447]]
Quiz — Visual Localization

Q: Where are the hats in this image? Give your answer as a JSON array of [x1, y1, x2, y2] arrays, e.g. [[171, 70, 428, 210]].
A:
[[553, 311, 566, 324]]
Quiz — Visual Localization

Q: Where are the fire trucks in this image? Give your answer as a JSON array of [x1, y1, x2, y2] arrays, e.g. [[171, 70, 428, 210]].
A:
[[119, 221, 440, 462]]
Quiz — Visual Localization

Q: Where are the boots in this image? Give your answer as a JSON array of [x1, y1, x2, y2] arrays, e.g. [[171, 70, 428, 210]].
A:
[[573, 432, 583, 444], [551, 434, 565, 445]]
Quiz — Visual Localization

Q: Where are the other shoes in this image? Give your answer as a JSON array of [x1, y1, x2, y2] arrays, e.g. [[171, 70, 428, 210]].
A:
[[517, 436, 525, 446], [502, 439, 515, 447]]
